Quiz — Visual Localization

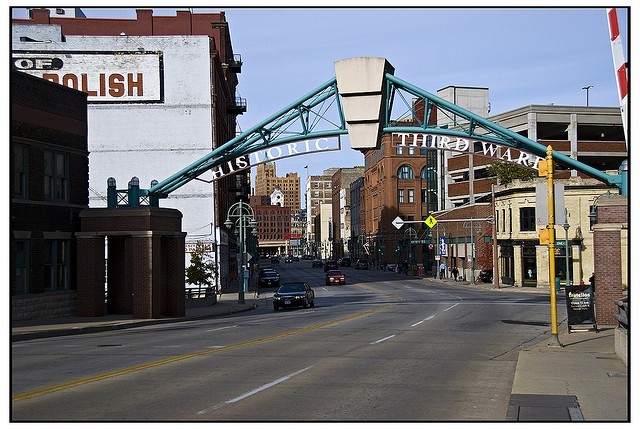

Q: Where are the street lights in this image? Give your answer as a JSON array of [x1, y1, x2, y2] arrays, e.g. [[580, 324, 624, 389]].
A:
[[587, 189, 612, 225], [223, 199, 258, 304], [428, 222, 446, 279], [357, 233, 367, 269], [234, 214, 256, 291], [404, 226, 417, 275], [463, 217, 482, 284]]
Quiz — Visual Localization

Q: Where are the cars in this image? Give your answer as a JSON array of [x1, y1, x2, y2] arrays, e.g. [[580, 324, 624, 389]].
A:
[[259, 272, 280, 288], [354, 259, 368, 270], [273, 282, 315, 311], [256, 259, 276, 276], [324, 259, 338, 272], [311, 259, 323, 269], [285, 257, 295, 263], [337, 258, 351, 267], [325, 270, 345, 285]]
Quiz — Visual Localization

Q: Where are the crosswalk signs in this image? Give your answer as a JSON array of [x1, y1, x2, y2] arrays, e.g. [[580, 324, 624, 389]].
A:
[[424, 215, 438, 229]]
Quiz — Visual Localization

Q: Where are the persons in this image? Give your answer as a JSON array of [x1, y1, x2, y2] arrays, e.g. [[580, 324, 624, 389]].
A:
[[440, 262, 447, 278], [451, 266, 460, 282], [402, 260, 412, 276]]
